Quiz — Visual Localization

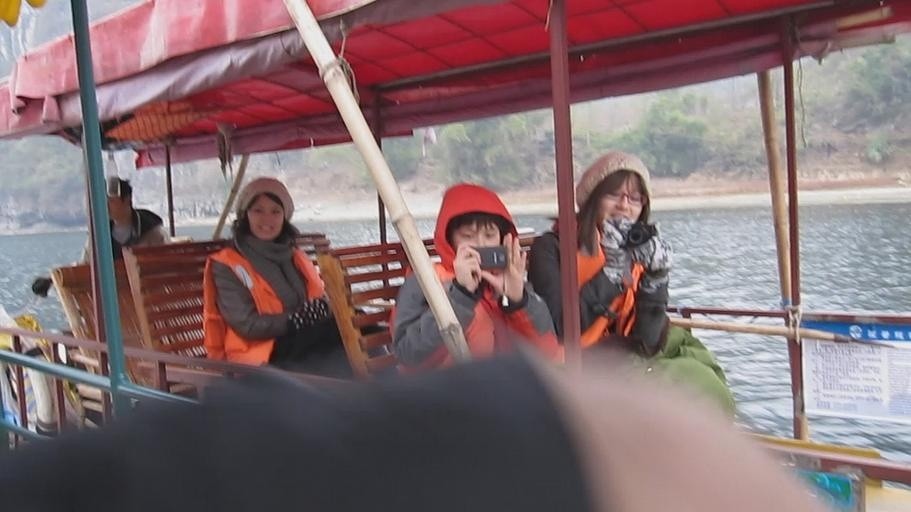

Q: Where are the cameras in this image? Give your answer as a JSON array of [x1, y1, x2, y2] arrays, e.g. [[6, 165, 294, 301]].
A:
[[620, 221, 658, 250], [473, 247, 508, 270]]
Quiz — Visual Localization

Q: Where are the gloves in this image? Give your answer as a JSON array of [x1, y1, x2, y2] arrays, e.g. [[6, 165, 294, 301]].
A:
[[599, 219, 633, 282], [287, 298, 329, 331], [636, 233, 673, 292]]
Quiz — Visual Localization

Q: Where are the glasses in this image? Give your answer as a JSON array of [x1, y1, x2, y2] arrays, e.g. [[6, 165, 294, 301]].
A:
[[606, 189, 647, 206]]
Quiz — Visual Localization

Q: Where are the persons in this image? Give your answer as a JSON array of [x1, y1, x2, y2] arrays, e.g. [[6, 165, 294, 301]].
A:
[[526, 151, 670, 371], [5, 353, 847, 509], [202, 176, 365, 380], [386, 184, 564, 383], [71, 179, 171, 270]]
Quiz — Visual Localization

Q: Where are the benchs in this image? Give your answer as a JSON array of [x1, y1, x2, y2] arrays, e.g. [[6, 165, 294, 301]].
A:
[[49, 227, 541, 428]]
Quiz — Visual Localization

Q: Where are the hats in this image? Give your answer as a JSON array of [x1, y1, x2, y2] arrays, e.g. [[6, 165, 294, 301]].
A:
[[576, 151, 651, 211], [235, 177, 294, 222]]
